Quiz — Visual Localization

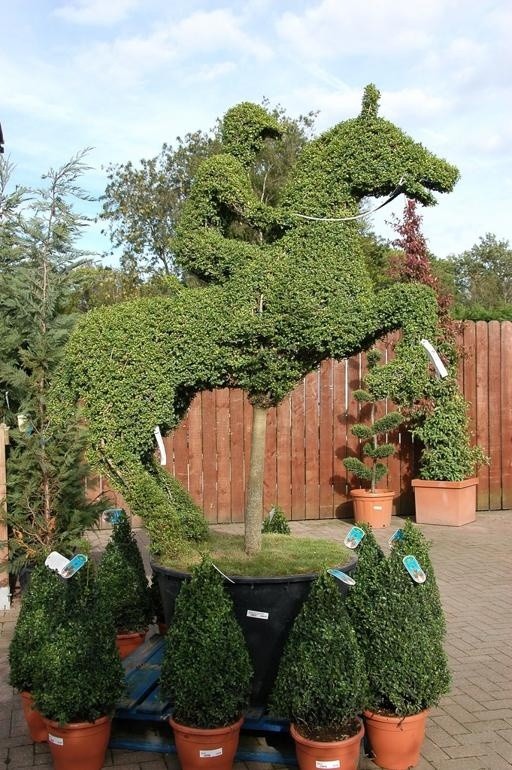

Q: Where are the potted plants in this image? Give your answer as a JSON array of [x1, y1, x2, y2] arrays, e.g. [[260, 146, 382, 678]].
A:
[[341, 375, 401, 529], [409, 360, 488, 528]]
